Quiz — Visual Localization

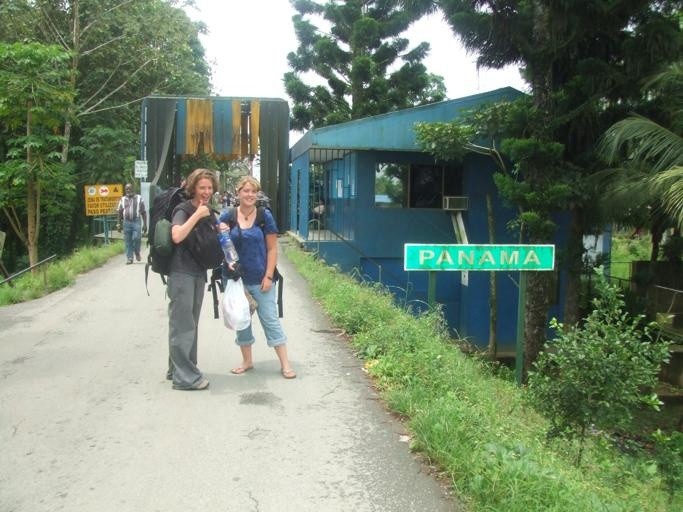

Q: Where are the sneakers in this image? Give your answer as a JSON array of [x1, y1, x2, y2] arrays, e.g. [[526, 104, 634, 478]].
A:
[[126, 251, 141, 264], [196, 378, 209, 389]]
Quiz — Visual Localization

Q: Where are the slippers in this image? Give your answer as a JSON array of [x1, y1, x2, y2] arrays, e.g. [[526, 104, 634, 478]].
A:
[[231, 366, 253, 374], [281, 367, 296, 378]]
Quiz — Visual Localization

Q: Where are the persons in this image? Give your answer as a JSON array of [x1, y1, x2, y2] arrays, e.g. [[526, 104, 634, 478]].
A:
[[116, 183, 147, 264], [220, 187, 237, 208], [165, 168, 231, 391], [296, 199, 325, 230], [218, 175, 296, 379]]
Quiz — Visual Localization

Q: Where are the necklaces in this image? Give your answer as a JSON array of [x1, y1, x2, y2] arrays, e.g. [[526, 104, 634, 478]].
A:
[[240, 206, 255, 221]]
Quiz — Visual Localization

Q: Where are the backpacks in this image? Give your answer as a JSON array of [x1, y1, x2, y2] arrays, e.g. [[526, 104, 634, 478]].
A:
[[227, 191, 271, 237], [149, 187, 225, 275]]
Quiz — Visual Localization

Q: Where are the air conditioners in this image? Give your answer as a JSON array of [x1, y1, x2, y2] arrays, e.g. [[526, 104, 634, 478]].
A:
[[442, 196, 470, 210]]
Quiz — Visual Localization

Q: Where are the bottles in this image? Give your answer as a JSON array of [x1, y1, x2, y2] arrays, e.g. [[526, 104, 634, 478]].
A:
[[215, 230, 239, 263]]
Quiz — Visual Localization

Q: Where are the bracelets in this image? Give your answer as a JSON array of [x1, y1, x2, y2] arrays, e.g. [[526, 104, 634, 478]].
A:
[[263, 274, 273, 281]]
